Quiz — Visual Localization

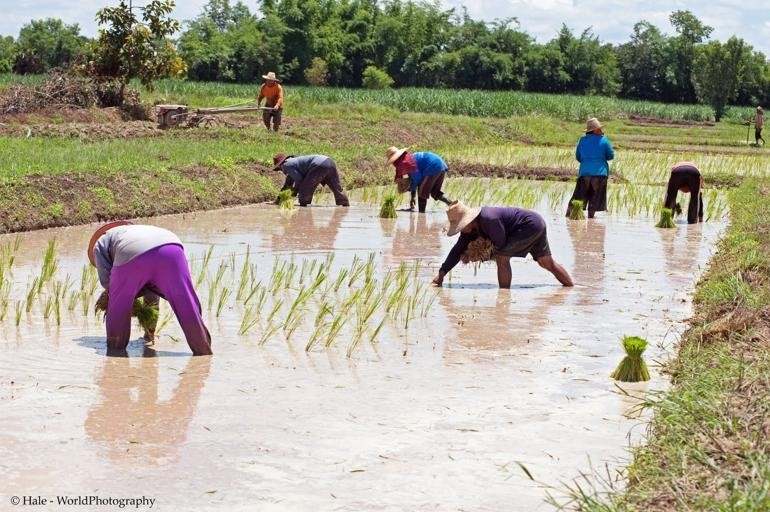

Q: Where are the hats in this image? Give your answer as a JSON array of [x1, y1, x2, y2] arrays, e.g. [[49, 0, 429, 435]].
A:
[[757, 106, 764, 113], [386, 145, 407, 167], [262, 71, 280, 83], [445, 200, 483, 236], [88, 221, 134, 266], [271, 152, 295, 171], [583, 117, 607, 133]]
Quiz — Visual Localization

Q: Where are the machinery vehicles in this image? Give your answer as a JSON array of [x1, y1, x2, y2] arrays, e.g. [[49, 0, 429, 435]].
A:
[[154, 102, 275, 129]]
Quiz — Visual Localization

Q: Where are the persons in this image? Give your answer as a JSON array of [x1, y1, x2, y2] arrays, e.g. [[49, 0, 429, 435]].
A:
[[391, 213, 448, 259], [663, 159, 705, 224], [662, 224, 703, 279], [257, 71, 285, 131], [267, 151, 350, 207], [84, 349, 216, 460], [87, 220, 214, 355], [429, 201, 574, 287], [564, 217, 608, 301], [385, 146, 456, 212], [435, 284, 572, 357], [272, 208, 350, 256], [749, 106, 766, 146], [566, 116, 615, 217]]
